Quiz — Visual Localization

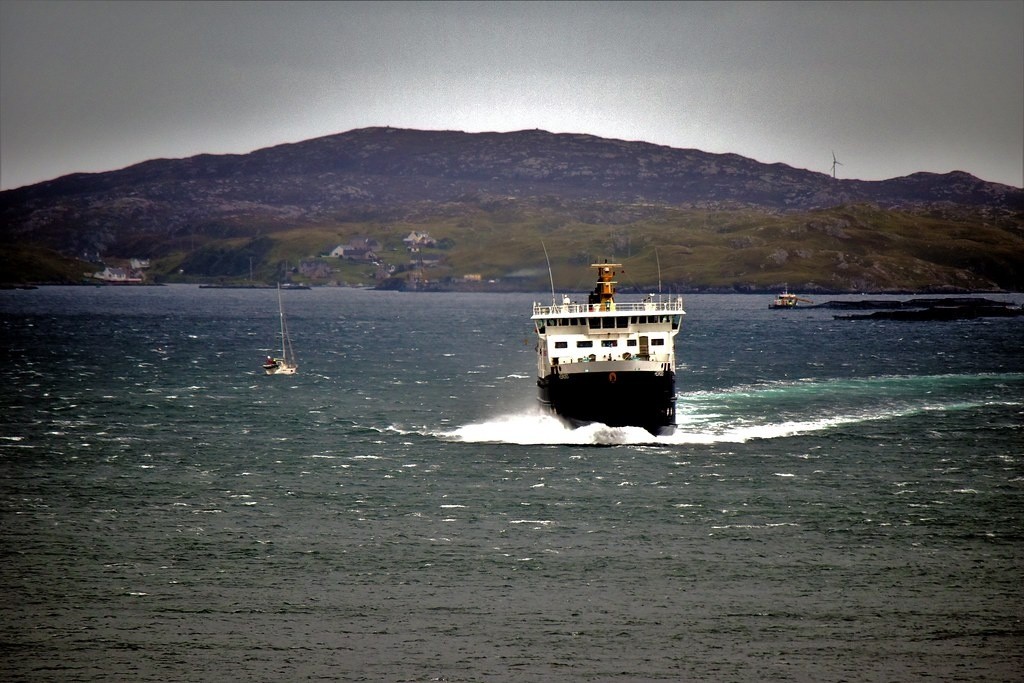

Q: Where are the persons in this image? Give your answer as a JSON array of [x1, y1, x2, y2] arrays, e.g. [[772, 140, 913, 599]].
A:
[[266, 356, 273, 365], [563, 295, 571, 306]]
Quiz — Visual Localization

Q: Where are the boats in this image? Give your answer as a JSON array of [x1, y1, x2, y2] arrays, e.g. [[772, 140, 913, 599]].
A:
[[768, 292, 798, 309], [530, 241, 687, 439]]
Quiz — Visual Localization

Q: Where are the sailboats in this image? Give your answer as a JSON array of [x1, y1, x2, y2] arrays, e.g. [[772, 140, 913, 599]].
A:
[[262, 280, 297, 374]]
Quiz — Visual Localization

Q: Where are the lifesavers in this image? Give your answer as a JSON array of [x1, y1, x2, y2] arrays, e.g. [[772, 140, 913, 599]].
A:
[[610, 372, 616, 381]]
